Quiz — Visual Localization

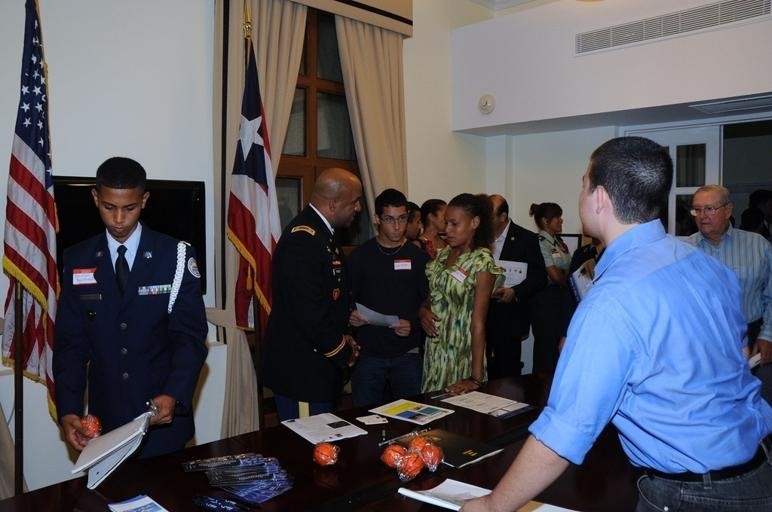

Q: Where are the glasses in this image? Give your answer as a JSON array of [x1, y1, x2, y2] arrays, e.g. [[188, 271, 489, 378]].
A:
[[376, 216, 406, 225], [689, 200, 730, 217]]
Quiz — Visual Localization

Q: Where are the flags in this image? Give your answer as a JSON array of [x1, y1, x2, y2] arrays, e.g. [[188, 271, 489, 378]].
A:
[[225, 25, 280, 331], [0, 0, 59, 427]]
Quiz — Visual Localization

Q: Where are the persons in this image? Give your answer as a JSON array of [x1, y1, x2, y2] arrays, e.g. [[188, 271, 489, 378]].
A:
[[670, 183, 772, 370], [255, 164, 362, 426], [456, 134, 771, 511], [52, 155, 210, 469], [739, 187, 772, 237]]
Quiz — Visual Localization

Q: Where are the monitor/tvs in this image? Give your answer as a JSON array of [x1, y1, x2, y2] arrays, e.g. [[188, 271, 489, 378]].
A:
[[51, 176, 208, 296]]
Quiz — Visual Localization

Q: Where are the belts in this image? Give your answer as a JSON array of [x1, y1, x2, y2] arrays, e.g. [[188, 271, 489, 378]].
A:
[[643, 430, 772, 483]]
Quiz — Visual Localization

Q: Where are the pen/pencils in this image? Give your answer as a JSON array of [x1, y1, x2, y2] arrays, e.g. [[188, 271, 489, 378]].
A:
[[378, 427, 431, 447]]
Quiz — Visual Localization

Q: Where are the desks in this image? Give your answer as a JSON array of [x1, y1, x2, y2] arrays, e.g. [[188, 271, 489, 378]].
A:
[[0, 372, 643, 511]]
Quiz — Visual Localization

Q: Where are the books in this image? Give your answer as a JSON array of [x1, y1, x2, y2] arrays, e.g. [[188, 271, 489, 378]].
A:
[[368, 398, 455, 426], [397, 478, 581, 512]]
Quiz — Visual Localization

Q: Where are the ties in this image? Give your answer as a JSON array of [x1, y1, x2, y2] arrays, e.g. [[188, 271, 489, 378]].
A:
[[115, 244, 131, 298]]
